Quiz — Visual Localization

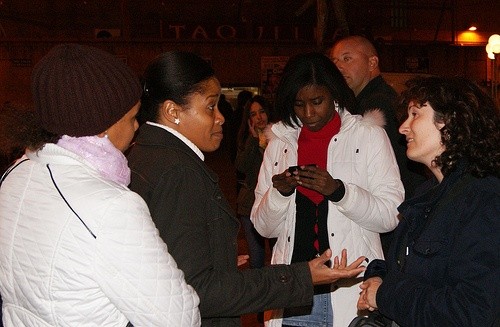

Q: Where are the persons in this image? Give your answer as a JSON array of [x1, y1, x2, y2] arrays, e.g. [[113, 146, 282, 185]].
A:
[[250, 50, 405, 327], [329, 36, 431, 260], [217, 90, 276, 272], [357, 74, 500, 327], [0, 42, 202, 327], [126, 50, 367, 327]]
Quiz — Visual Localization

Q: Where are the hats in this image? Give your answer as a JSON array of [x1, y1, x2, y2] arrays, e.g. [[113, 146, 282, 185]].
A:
[[30, 43, 141, 137]]
